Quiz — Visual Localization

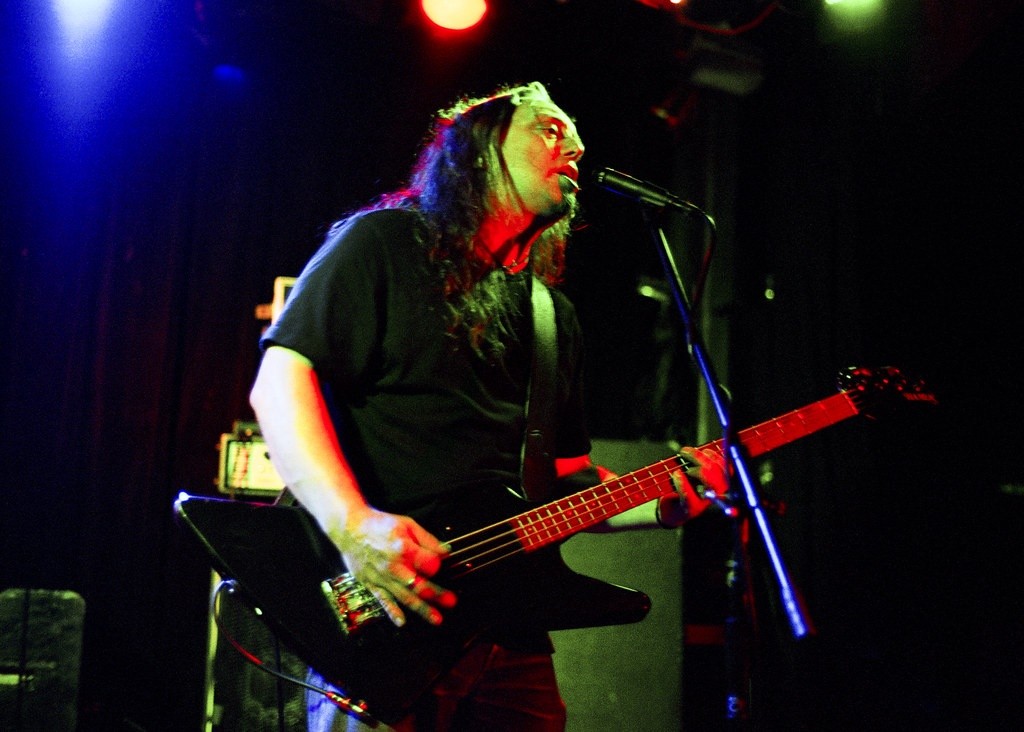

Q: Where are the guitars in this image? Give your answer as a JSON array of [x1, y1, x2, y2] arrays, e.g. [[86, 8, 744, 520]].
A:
[[170, 368, 942, 723]]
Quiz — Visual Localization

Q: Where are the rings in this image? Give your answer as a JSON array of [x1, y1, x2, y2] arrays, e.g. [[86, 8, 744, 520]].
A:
[[406, 573, 420, 590]]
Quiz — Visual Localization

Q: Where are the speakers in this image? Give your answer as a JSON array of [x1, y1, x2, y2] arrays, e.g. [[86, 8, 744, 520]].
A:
[[207, 569, 313, 731]]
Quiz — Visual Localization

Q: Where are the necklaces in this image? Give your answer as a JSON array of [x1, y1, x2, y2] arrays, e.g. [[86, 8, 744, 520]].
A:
[[476, 234, 529, 273]]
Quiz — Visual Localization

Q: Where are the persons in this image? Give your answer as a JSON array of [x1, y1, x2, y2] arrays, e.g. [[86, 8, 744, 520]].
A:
[[249, 82, 734, 732]]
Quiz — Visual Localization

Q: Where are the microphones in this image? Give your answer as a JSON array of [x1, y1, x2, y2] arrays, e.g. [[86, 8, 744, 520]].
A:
[[598, 167, 705, 219]]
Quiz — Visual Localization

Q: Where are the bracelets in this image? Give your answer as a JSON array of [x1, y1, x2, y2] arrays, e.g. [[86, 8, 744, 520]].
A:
[[655, 498, 678, 530]]
